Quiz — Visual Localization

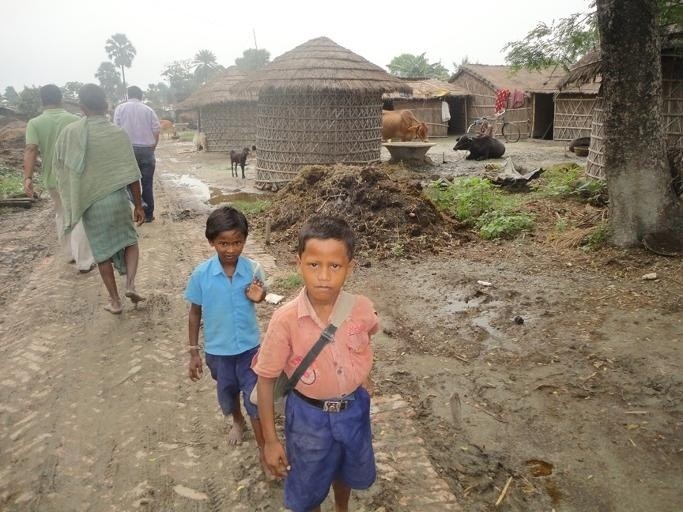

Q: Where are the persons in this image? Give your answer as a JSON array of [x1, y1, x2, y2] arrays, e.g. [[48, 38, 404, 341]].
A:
[[23, 83, 96, 274], [249, 212, 381, 512], [182, 205, 284, 485], [111, 85, 160, 224], [49, 80, 146, 314]]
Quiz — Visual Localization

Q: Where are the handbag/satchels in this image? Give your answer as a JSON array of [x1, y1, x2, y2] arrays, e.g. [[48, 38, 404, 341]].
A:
[[273, 371, 292, 402]]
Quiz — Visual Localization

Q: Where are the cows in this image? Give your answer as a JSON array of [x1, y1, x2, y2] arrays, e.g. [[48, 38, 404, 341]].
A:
[[158, 119, 176, 140], [453, 134, 506, 161], [381, 108, 430, 143]]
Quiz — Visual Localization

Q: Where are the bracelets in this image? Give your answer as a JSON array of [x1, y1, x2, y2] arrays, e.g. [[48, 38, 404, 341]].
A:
[[22, 176, 31, 182], [186, 343, 201, 351]]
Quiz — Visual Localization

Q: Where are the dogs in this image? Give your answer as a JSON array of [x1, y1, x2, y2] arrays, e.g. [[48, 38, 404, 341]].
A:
[[229, 148, 251, 173]]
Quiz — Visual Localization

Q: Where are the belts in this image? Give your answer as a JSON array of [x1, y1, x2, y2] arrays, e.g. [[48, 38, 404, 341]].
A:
[[293, 388, 351, 412]]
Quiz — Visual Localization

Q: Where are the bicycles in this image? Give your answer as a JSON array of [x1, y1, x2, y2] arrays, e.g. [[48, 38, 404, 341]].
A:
[[466, 107, 520, 143]]
